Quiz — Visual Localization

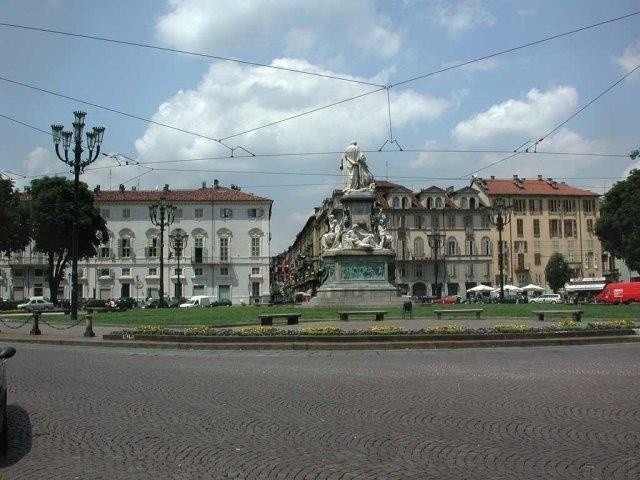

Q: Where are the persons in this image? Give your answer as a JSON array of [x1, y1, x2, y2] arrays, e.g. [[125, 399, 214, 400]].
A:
[[411, 289, 600, 304], [318, 141, 398, 252], [56, 294, 186, 313]]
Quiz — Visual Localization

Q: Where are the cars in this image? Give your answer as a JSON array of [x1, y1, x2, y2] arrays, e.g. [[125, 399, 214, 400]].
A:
[[433, 296, 460, 304], [210, 299, 232, 307], [17, 299, 55, 311], [81, 297, 138, 311], [142, 300, 188, 309]]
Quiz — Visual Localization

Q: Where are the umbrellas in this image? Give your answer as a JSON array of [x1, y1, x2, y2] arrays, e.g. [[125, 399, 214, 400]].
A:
[[519, 283, 546, 296], [492, 284, 523, 296], [467, 284, 495, 297]]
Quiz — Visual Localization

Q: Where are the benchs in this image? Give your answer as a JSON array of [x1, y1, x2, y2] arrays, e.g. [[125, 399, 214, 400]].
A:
[[338, 311, 388, 322], [434, 309, 486, 319], [258, 313, 301, 326], [532, 310, 584, 322]]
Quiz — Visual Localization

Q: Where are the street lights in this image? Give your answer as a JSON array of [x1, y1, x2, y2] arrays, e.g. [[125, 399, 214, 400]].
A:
[[426, 227, 446, 295], [148, 198, 188, 298], [50, 110, 106, 321], [486, 197, 514, 300]]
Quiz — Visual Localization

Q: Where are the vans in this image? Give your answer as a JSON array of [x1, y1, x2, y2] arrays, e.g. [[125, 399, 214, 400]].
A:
[[497, 295, 528, 304], [528, 294, 565, 304], [596, 282, 640, 305]]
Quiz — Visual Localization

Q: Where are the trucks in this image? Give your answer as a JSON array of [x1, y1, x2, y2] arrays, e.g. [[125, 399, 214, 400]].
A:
[[180, 296, 210, 308]]
